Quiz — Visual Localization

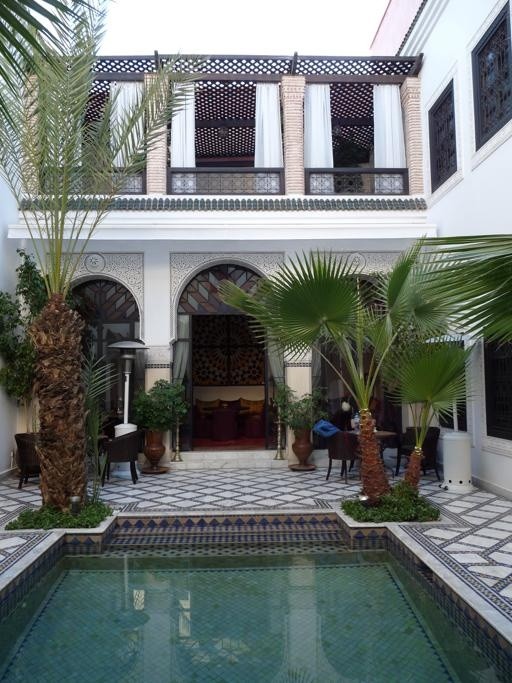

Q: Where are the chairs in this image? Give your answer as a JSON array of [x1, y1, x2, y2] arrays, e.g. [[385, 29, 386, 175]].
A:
[[195, 397, 265, 436], [101, 428, 144, 486], [14, 432, 41, 489], [326, 431, 358, 483], [395, 425, 441, 481]]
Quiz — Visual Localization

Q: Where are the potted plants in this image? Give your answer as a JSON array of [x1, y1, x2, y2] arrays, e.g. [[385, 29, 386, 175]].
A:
[[268, 372, 331, 472], [133, 376, 191, 474]]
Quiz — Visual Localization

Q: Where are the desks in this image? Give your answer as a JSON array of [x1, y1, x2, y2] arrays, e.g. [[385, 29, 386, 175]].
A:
[[210, 407, 238, 440], [352, 428, 402, 476]]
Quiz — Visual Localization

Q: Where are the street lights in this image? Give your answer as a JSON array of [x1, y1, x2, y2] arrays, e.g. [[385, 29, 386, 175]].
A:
[[110, 555, 150, 629]]
